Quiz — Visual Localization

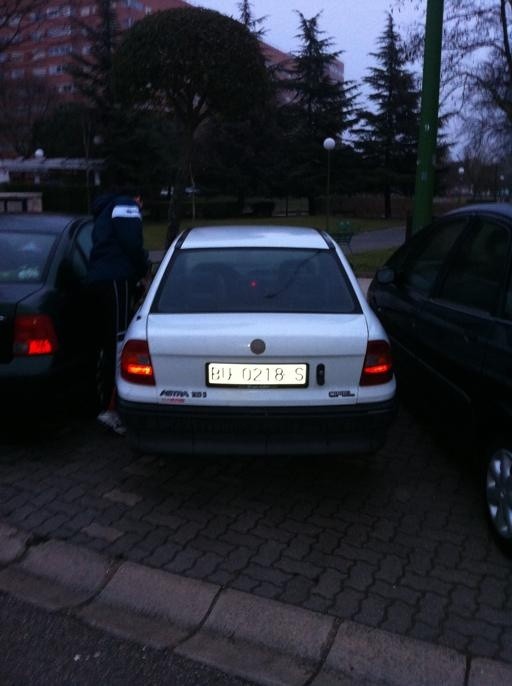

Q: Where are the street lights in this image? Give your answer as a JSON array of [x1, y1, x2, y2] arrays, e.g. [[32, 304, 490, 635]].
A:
[[456, 168, 466, 205], [323, 138, 335, 233]]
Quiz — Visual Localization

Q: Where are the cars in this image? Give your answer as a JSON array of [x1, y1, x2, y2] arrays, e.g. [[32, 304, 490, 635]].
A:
[[0, 213, 96, 438], [117, 224, 399, 459], [369, 203, 511, 555]]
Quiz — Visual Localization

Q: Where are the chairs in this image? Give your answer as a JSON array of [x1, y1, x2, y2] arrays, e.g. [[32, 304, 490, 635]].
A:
[[190, 259, 315, 298]]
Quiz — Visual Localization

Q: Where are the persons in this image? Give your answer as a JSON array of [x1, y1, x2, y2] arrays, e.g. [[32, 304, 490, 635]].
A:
[[87, 179, 154, 434]]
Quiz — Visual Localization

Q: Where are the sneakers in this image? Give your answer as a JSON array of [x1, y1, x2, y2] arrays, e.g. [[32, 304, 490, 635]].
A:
[[97, 411, 128, 436]]
